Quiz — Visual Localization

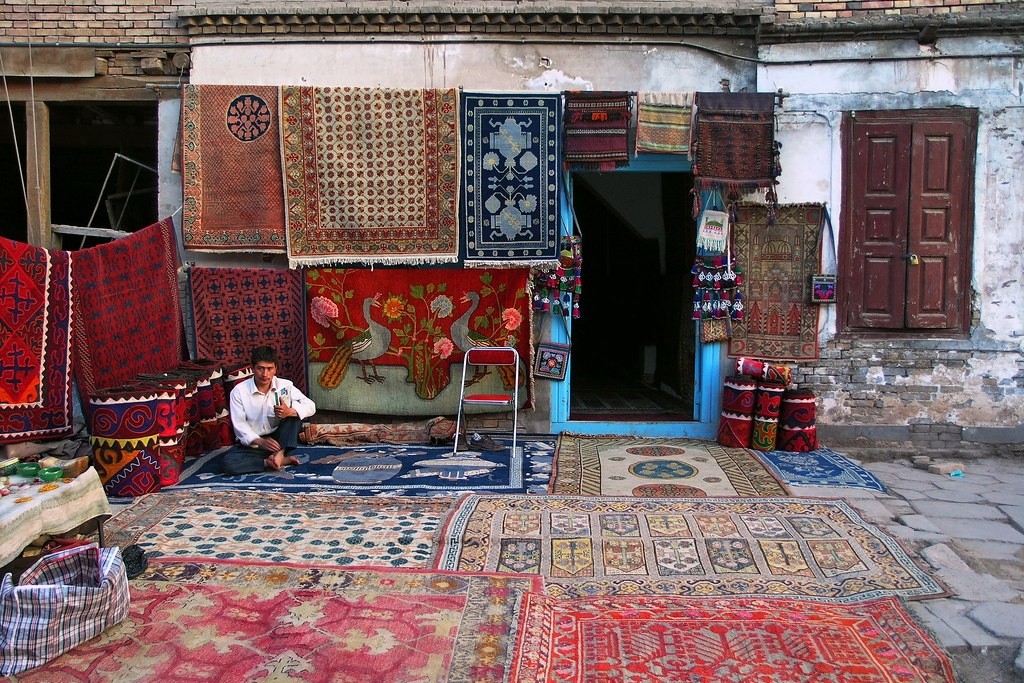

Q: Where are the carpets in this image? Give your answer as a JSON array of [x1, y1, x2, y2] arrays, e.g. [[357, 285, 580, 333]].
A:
[[0, 87, 961, 683]]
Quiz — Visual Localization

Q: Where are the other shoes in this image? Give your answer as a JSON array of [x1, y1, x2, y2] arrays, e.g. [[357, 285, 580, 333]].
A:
[[471, 435, 505, 451]]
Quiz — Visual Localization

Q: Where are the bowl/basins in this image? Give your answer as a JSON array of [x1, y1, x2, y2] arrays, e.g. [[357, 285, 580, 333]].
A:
[[15, 462, 39, 477], [0, 461, 20, 476], [37, 467, 64, 482]]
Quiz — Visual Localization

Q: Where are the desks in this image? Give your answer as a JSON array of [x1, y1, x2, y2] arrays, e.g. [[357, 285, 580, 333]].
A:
[[0, 466, 112, 583]]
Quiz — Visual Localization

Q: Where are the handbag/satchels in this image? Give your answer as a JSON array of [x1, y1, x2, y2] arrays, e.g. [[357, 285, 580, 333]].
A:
[[561, 250, 574, 268], [700, 316, 732, 342], [557, 236, 582, 272], [534, 341, 570, 379], [811, 274, 837, 302], [697, 190, 729, 251], [1, 542, 130, 676]]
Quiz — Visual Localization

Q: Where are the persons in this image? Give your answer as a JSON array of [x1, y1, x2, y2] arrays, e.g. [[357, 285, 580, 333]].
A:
[[222, 345, 315, 475]]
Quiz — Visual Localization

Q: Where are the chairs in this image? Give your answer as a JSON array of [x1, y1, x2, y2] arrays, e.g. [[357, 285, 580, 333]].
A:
[[452, 347, 519, 457]]
